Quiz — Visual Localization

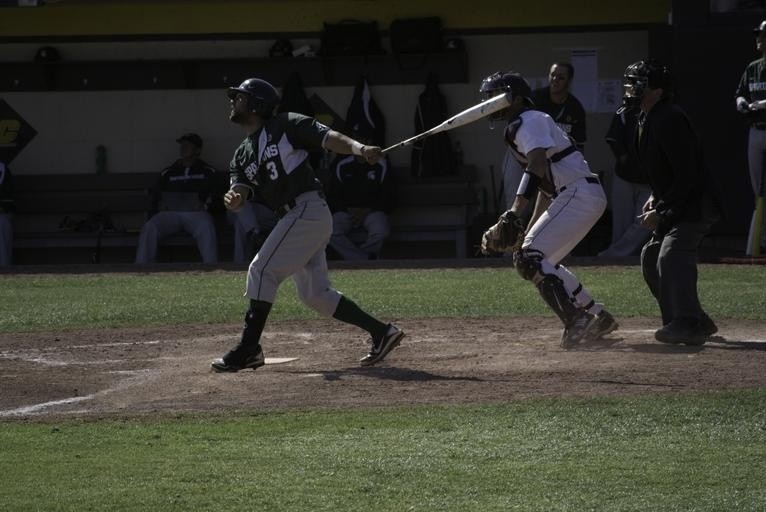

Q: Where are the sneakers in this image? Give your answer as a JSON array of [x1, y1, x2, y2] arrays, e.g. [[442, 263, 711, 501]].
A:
[[680, 310, 717, 337], [358, 324, 406, 367], [555, 310, 597, 349], [654, 313, 705, 346], [577, 310, 620, 345], [210, 345, 264, 372]]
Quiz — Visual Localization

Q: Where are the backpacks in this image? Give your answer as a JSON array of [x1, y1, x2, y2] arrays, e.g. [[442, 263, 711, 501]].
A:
[[412, 76, 460, 176]]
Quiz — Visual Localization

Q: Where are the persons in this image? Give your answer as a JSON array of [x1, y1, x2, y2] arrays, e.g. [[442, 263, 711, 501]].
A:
[[330, 123, 396, 260], [211, 77, 406, 371], [617, 58, 721, 348], [735, 18, 766, 260], [0, 158, 15, 266], [477, 69, 622, 350], [499, 60, 587, 266], [226, 187, 281, 265], [135, 131, 223, 269]]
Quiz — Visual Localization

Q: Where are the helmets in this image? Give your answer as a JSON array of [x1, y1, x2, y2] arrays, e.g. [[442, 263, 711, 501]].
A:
[[618, 57, 671, 113], [226, 77, 279, 107], [478, 70, 536, 119]]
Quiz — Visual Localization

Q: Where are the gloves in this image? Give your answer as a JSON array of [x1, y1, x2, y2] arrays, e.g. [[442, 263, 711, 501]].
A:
[[749, 98, 766, 111], [736, 96, 749, 114]]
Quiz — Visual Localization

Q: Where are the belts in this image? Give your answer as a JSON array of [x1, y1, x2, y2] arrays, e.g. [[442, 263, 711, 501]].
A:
[[261, 189, 327, 218], [547, 176, 601, 197]]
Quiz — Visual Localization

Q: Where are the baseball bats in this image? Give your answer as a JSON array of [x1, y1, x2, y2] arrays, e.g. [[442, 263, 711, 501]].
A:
[[382, 93, 512, 153]]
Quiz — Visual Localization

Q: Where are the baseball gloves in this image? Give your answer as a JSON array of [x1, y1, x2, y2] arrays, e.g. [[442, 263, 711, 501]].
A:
[[481, 211, 524, 255]]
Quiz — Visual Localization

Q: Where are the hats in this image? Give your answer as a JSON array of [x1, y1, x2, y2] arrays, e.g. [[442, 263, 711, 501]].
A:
[[750, 19, 766, 33], [175, 132, 203, 146]]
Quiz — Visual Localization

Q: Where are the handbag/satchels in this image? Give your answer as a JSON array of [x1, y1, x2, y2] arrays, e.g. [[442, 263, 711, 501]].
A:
[[320, 15, 384, 53], [335, 75, 389, 184], [389, 16, 451, 54]]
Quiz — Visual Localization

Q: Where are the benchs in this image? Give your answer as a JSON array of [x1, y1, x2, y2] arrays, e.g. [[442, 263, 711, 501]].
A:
[[11, 170, 480, 257]]
